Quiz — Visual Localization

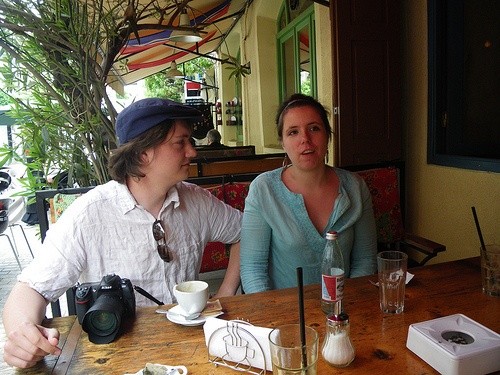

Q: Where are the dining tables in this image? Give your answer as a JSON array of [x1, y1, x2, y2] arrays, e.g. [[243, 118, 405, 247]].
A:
[[1, 254, 500, 375]]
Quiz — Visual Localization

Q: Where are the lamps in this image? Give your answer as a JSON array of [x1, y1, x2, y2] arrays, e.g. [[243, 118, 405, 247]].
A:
[[169, 9, 202, 43], [165, 60, 185, 79]]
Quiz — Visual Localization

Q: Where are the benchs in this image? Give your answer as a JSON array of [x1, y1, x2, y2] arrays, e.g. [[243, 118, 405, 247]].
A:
[[36, 146, 445, 319]]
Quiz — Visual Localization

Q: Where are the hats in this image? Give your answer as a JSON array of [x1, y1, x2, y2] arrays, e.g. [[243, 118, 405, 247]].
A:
[[115, 97, 204, 143]]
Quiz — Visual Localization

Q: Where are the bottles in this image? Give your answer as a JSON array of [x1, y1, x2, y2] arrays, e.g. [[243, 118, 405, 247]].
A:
[[320, 312, 356, 369], [320, 229, 346, 314]]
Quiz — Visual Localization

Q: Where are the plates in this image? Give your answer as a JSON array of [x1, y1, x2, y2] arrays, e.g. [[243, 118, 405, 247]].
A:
[[135, 364, 188, 375], [167, 304, 224, 327], [232, 97, 238, 104], [230, 116, 237, 121]]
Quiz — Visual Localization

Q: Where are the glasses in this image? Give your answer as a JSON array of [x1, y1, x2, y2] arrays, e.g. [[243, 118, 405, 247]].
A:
[[152, 219, 173, 263]]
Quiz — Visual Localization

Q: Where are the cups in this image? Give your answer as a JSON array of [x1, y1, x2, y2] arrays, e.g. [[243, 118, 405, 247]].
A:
[[480, 244, 500, 296], [268, 324, 319, 375], [378, 250, 408, 315], [173, 280, 209, 315]]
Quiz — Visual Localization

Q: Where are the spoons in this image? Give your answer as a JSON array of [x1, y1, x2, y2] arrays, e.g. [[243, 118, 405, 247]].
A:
[[156, 310, 201, 321]]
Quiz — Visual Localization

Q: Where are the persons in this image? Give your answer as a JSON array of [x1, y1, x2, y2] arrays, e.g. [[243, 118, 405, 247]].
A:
[[3, 98, 247, 369], [239, 93, 377, 295]]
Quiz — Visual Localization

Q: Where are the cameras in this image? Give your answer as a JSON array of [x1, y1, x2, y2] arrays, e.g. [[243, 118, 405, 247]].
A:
[[74, 274, 136, 344]]
